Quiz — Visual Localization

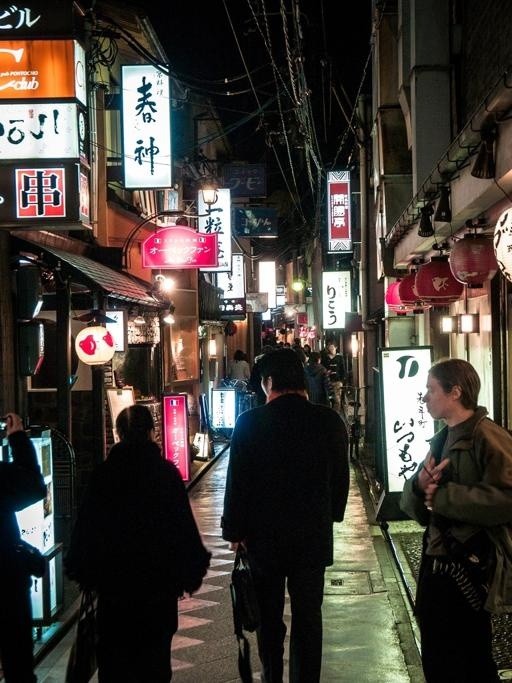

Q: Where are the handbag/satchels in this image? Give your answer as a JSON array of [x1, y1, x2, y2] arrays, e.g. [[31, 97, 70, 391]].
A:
[[66, 615, 98, 683], [230, 568, 261, 633]]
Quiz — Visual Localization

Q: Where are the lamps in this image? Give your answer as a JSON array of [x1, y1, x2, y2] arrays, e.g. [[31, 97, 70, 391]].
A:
[[439, 313, 482, 337], [72, 311, 116, 366], [180, 179, 219, 219], [134, 305, 176, 324]]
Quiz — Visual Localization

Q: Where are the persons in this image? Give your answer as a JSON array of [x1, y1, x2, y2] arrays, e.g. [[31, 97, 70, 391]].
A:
[[228, 330, 348, 409], [218, 346, 350, 682], [400, 358, 512, 682], [64, 404, 214, 681], [0, 409, 54, 682]]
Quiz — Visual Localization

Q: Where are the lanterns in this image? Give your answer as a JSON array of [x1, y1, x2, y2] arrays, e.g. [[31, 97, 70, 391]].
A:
[[398, 271, 433, 314], [448, 233, 501, 289], [493, 207, 512, 286], [385, 280, 410, 316], [74, 324, 116, 367], [415, 256, 464, 308]]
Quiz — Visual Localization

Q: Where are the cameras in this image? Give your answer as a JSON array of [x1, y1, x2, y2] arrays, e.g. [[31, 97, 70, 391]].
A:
[[0, 418, 8, 440]]
